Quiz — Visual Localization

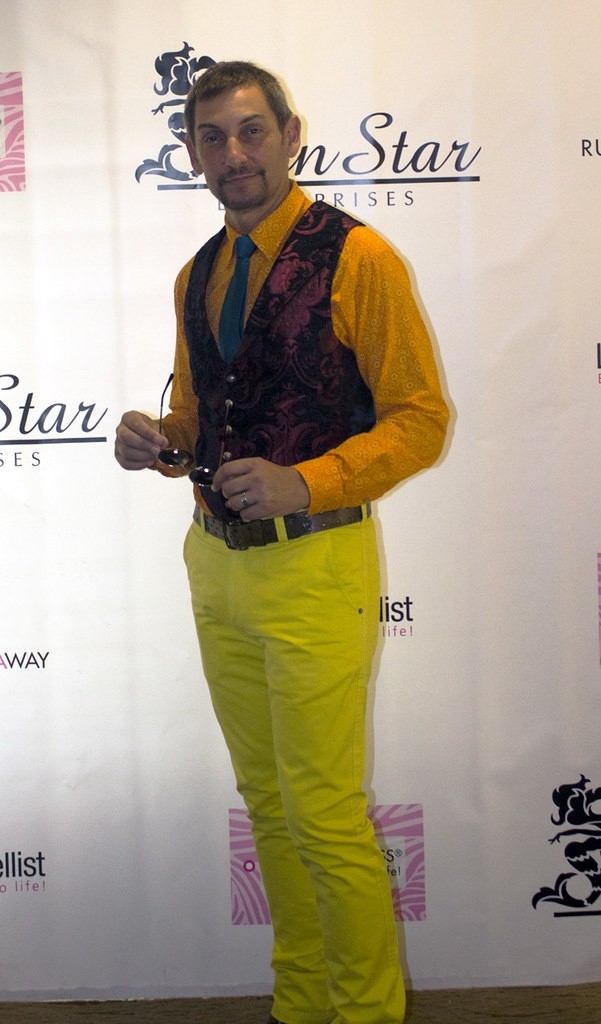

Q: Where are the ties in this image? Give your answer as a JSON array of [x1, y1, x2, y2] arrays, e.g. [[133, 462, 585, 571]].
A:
[[218, 235, 257, 366]]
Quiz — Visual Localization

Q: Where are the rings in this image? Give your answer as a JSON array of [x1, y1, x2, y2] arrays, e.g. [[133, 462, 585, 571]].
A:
[[240, 492, 250, 506]]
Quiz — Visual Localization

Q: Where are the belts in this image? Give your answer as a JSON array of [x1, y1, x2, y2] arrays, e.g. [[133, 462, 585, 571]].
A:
[[192, 502, 371, 551]]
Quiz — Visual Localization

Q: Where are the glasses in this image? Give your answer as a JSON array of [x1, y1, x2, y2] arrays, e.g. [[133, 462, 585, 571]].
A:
[[156, 376, 232, 486]]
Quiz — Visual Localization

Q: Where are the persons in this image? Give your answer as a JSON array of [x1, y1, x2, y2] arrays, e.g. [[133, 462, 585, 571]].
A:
[[116, 62, 455, 1023]]
